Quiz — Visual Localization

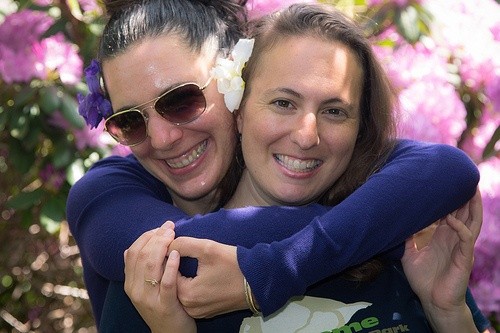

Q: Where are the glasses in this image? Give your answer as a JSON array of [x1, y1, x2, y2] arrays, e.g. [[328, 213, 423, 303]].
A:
[[104, 74, 213, 148]]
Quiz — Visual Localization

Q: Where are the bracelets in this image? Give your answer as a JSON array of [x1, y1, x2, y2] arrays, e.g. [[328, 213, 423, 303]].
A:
[[241, 274, 263, 318]]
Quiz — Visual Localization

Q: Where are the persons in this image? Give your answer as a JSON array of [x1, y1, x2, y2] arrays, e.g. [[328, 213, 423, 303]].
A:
[[122, 1, 479, 332], [64, 0, 481, 333]]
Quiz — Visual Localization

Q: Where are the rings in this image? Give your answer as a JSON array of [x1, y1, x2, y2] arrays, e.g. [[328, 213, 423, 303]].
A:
[[142, 275, 163, 286]]
